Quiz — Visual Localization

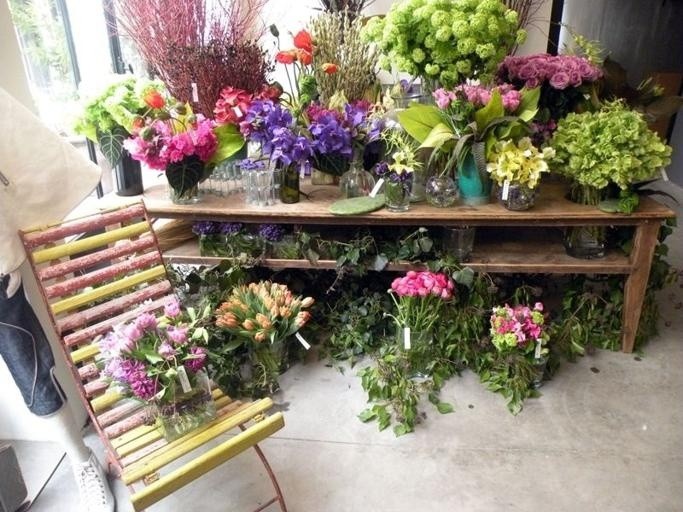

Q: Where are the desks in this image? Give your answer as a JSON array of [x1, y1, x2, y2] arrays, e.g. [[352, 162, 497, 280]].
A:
[[94, 172, 676, 357]]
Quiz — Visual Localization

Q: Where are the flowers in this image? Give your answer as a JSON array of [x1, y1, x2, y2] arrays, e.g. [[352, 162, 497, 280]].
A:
[[86, 315, 207, 413], [207, 282, 313, 344], [488, 298, 549, 356], [381, 272, 455, 319], [96, 4, 603, 188]]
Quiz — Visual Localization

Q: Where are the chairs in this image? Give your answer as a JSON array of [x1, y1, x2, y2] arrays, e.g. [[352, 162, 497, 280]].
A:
[[15, 197, 291, 511]]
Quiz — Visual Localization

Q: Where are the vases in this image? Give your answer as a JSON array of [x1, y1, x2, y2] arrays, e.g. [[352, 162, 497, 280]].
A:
[[144, 380, 219, 442], [502, 348, 556, 396], [114, 93, 614, 264], [250, 341, 296, 380], [393, 316, 430, 358]]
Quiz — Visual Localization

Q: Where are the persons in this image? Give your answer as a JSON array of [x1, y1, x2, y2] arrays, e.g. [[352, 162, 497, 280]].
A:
[[0, 266, 117, 511]]
